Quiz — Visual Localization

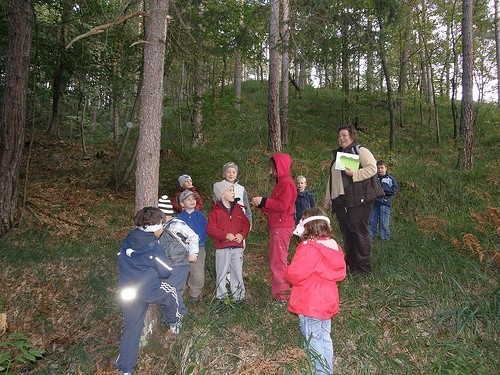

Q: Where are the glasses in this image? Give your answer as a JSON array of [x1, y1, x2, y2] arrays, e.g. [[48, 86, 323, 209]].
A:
[[338, 134, 349, 138]]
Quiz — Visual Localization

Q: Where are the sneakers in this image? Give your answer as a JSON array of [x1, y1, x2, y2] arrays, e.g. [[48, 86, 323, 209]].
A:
[[170, 314, 183, 334]]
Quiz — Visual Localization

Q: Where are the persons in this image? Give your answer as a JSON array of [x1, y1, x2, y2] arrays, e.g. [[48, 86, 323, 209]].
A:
[[115, 206, 183, 375], [205, 180, 250, 313], [176, 190, 208, 307], [251, 154, 298, 307], [171, 174, 203, 214], [158, 195, 199, 317], [283, 206, 346, 374], [322, 124, 386, 278], [213, 161, 253, 285], [294, 175, 316, 228], [369, 160, 398, 242]]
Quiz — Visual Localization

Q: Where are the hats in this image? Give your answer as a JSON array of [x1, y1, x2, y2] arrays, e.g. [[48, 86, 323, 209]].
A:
[[157, 195, 174, 214], [223, 162, 239, 174], [213, 181, 234, 201], [178, 174, 191, 187], [179, 190, 196, 203]]
